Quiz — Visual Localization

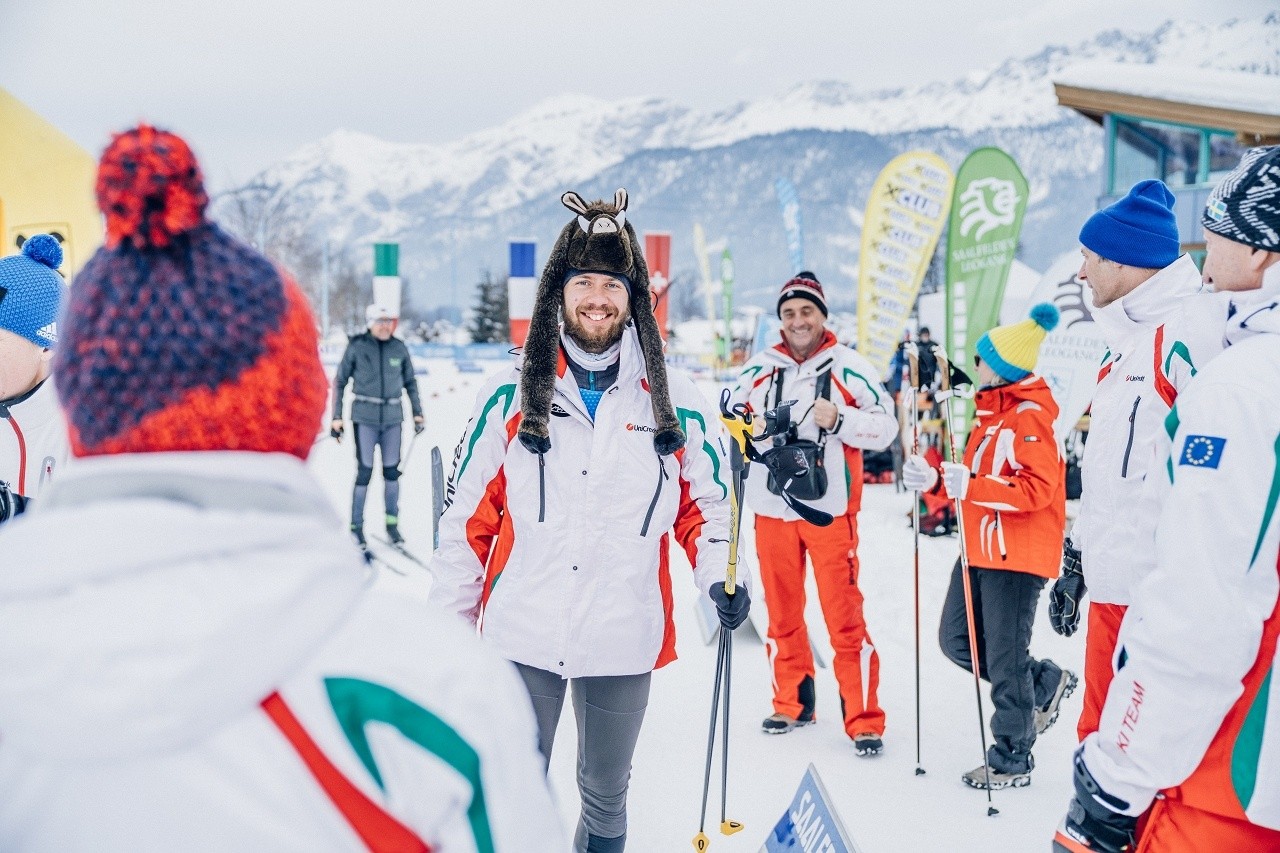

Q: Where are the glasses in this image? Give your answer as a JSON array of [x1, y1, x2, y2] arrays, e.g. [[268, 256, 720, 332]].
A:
[[974, 356, 982, 366]]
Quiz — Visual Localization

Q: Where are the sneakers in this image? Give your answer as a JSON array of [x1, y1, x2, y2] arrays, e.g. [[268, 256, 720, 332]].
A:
[[854, 732, 884, 756], [962, 765, 1031, 789], [1035, 669, 1078, 735], [762, 712, 817, 734]]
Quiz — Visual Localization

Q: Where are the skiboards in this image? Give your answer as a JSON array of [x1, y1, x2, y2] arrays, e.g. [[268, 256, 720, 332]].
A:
[[370, 533, 430, 577], [888, 377, 907, 493]]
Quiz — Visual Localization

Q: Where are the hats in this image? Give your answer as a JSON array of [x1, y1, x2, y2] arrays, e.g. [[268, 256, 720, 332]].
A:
[[776, 271, 828, 320], [1079, 179, 1180, 268], [1200, 146, 1280, 252], [976, 303, 1060, 383], [562, 269, 632, 298], [0, 233, 68, 350], [53, 124, 329, 459], [518, 188, 686, 454]]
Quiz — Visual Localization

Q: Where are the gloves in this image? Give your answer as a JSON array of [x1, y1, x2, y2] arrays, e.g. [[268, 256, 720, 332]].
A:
[[941, 461, 970, 500], [414, 415, 424, 434], [902, 455, 938, 493], [331, 419, 344, 443], [1052, 744, 1135, 853], [1049, 537, 1088, 637], [709, 581, 751, 630]]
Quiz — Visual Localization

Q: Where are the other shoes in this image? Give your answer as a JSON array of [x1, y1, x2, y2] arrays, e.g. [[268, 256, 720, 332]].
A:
[[387, 524, 405, 548], [351, 528, 367, 550]]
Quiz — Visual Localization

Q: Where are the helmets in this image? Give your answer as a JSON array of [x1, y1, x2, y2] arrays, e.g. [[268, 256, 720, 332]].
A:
[[366, 303, 400, 329]]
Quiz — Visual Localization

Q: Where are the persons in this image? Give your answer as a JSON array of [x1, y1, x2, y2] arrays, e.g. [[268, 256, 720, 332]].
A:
[[0, 127, 1280, 853], [719, 271, 900, 757]]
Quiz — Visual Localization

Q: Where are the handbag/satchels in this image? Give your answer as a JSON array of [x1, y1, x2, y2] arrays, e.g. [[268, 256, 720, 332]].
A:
[[766, 439, 828, 500]]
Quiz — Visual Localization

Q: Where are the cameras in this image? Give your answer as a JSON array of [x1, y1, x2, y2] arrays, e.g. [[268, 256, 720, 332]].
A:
[[772, 421, 799, 446]]
